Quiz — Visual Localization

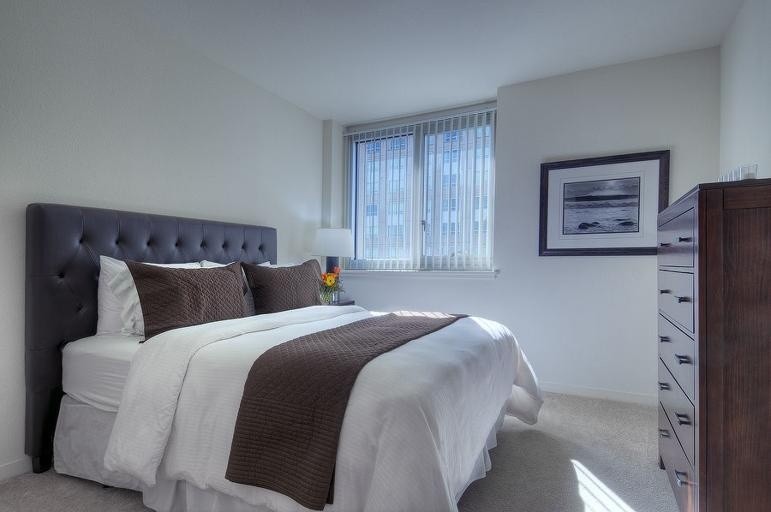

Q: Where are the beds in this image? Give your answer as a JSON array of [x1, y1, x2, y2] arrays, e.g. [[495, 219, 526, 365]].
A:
[[24, 203, 543, 512]]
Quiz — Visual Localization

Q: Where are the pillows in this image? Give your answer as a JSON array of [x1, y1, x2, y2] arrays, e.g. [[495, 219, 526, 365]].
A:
[[85, 255, 251, 343], [240, 256, 325, 315]]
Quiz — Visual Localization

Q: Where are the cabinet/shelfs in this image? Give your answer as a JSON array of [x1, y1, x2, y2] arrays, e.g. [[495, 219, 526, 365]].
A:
[[659, 179, 771, 511]]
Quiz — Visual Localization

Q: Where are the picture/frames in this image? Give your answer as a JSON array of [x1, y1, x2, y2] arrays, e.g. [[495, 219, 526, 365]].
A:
[[539, 150, 670, 257]]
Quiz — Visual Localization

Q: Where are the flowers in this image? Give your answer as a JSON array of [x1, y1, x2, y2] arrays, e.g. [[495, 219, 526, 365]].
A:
[[318, 267, 345, 306]]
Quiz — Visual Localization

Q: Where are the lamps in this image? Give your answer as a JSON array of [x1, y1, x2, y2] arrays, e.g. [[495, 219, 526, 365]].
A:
[[309, 229, 355, 302]]
